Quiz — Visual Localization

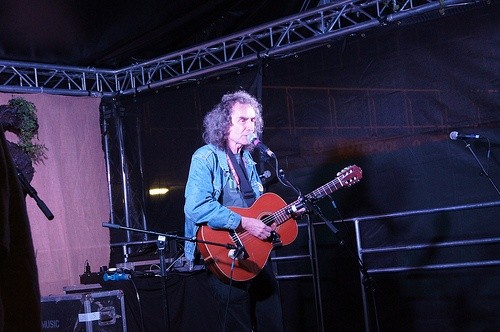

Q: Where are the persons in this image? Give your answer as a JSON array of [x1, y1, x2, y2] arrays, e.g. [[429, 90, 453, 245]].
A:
[[184, 92, 307, 332]]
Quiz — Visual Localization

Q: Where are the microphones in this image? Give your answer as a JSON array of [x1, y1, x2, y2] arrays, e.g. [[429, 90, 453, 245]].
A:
[[247, 133, 276, 158], [258, 170, 271, 178], [228, 246, 245, 260], [449, 131, 487, 140]]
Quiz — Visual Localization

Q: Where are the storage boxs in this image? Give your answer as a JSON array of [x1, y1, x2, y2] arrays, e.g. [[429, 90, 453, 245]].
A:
[[40, 289, 126, 332]]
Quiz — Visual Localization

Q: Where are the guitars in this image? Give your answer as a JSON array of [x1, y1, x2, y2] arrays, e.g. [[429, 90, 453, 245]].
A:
[[195, 163, 364, 284]]
